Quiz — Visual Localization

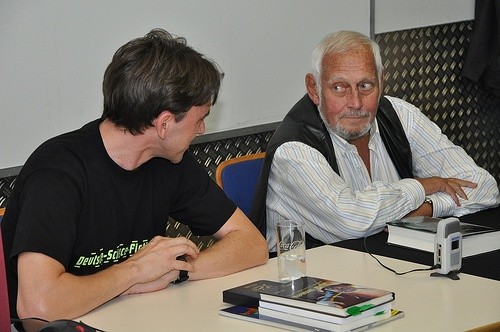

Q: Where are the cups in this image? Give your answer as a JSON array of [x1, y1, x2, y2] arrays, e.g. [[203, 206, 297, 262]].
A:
[[276, 220, 307, 284]]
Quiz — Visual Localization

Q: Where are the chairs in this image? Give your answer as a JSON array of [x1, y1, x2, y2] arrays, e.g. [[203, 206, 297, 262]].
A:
[[215, 152, 268, 239]]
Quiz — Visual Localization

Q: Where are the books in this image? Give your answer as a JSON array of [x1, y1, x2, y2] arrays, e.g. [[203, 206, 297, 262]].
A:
[[220, 276, 405, 332], [385, 219, 500, 260]]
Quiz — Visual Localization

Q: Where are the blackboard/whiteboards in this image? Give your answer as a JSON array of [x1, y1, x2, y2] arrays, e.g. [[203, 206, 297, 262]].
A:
[[0, 0, 376, 182], [374, 0, 476, 34]]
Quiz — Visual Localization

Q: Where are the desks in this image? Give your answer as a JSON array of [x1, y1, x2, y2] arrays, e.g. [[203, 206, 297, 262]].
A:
[[10, 204, 500, 332]]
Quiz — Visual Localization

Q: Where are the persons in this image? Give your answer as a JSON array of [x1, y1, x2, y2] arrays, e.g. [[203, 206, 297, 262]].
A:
[[252, 31, 500, 252], [0, 28, 269, 322]]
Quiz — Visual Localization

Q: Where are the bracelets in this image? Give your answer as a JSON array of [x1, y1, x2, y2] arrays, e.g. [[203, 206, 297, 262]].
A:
[[424, 198, 434, 219], [172, 257, 190, 285]]
